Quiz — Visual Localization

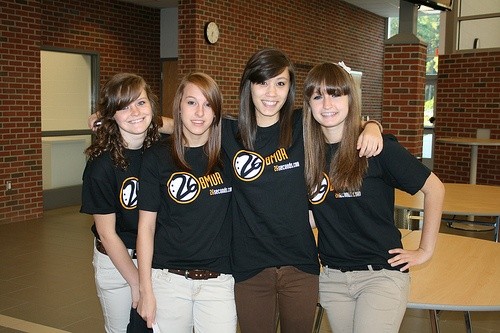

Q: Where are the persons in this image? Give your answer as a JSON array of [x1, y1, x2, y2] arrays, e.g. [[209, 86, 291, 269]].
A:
[[303, 62, 445, 333], [136, 73, 237, 333], [87, 49, 384, 333], [92, 73, 173, 333]]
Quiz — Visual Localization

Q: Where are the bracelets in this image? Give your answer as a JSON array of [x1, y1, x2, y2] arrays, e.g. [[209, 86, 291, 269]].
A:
[[362, 119, 382, 132]]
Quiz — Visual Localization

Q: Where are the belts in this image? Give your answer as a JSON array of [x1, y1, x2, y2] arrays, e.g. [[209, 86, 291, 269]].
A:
[[152, 265, 220, 280], [96, 239, 137, 259], [322, 264, 400, 273]]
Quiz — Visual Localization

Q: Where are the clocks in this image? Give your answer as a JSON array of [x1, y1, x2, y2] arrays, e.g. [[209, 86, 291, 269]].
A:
[[204, 21, 219, 45]]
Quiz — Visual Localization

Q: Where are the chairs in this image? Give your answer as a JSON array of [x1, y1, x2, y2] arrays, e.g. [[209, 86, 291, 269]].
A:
[[445, 215, 500, 242]]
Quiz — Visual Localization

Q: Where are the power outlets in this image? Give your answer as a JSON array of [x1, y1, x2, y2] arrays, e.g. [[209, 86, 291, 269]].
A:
[[7, 181, 12, 190]]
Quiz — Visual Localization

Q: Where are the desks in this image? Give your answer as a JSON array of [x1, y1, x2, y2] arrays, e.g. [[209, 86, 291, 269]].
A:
[[436, 137, 500, 220], [313, 227, 500, 333], [393, 182, 500, 242]]
